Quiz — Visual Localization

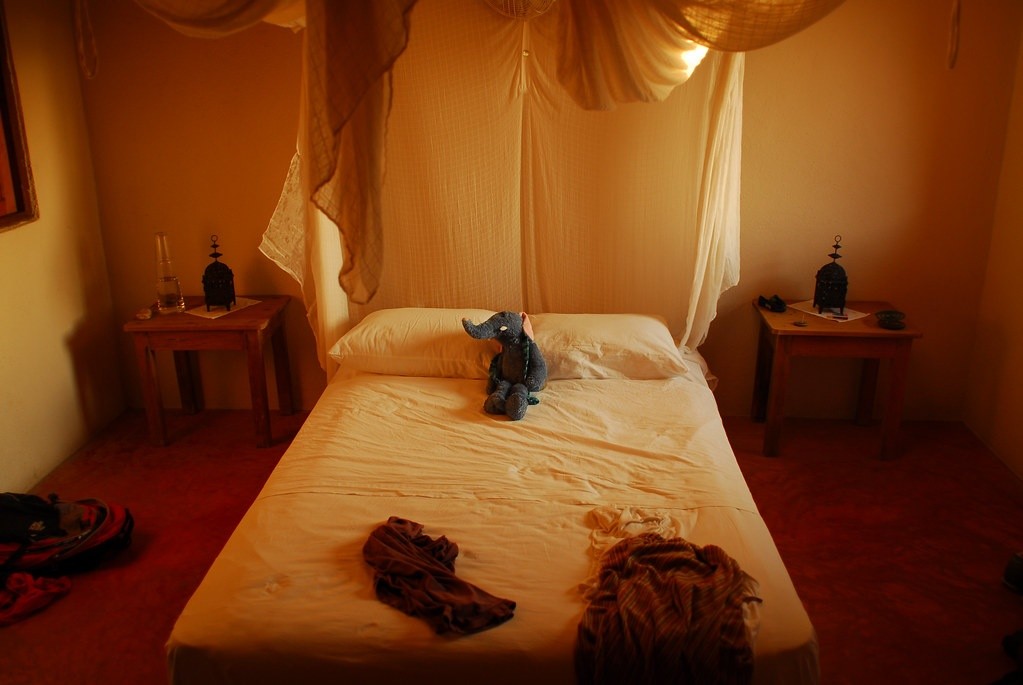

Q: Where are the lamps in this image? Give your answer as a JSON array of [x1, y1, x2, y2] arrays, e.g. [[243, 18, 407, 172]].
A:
[[201, 234, 235, 311], [812, 235, 849, 316]]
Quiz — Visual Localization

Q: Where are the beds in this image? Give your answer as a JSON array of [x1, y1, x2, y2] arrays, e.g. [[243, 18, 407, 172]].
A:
[[167, 363, 825, 684]]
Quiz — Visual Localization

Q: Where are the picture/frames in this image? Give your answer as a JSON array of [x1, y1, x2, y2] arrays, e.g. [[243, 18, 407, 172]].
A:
[[0, 0, 41, 236]]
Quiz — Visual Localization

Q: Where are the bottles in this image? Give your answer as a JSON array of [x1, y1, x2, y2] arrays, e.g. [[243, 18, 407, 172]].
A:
[[155, 232, 186, 316]]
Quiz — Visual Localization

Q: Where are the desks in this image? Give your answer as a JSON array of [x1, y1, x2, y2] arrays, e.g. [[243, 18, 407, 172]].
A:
[[753, 298, 924, 461], [123, 294, 294, 448]]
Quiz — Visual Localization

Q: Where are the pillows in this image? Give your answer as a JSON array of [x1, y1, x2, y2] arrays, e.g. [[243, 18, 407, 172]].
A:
[[327, 308, 501, 381], [526, 314, 687, 382]]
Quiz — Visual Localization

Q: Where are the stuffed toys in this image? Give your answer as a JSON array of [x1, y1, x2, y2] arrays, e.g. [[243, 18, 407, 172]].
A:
[[462, 311, 547, 420]]
